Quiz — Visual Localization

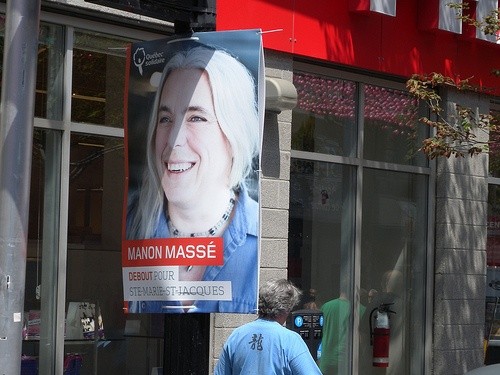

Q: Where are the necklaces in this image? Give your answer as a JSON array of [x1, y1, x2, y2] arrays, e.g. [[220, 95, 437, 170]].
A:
[[167, 190, 239, 272]]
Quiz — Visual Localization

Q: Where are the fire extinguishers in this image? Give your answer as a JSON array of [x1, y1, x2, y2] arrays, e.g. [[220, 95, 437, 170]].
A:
[[370, 303, 398, 368]]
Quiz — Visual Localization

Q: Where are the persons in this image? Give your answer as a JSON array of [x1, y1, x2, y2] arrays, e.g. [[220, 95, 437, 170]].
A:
[[125, 44, 259, 313], [298, 269, 405, 375], [214, 278, 322, 375]]
